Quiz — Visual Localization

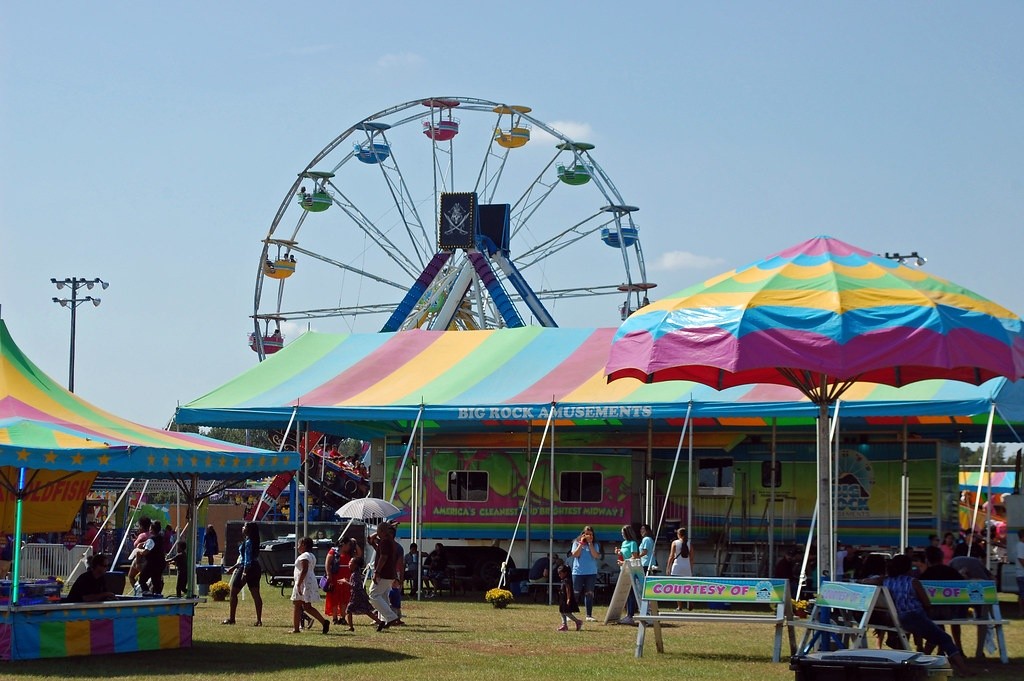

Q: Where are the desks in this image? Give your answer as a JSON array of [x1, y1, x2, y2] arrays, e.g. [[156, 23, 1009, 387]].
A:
[[281, 563, 367, 597]]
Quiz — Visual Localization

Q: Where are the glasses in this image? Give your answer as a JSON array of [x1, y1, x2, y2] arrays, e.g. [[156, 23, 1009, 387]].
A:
[[934, 539, 940, 541]]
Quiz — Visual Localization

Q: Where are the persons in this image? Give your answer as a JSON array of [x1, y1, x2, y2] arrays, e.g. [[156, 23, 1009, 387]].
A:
[[622, 298, 650, 317], [773, 503, 1024, 681], [66, 515, 218, 602], [528, 522, 694, 631], [265, 253, 299, 338], [290, 529, 457, 635], [221, 521, 263, 626], [313, 443, 370, 480]]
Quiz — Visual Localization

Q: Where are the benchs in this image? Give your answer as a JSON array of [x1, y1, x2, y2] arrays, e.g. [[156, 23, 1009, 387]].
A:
[[528, 582, 564, 604], [274, 575, 326, 597], [632, 576, 1010, 664]]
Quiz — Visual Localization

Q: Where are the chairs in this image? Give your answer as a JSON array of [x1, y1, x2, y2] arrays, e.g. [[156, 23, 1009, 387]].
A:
[[582, 569, 612, 605], [402, 564, 474, 597]]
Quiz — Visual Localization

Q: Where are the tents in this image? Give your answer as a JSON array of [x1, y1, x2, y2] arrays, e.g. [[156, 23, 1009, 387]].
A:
[[1, 303, 301, 607], [174, 324, 1024, 616]]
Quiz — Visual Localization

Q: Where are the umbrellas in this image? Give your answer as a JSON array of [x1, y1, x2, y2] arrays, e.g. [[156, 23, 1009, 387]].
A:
[[335, 497, 401, 545], [602, 236, 1024, 592]]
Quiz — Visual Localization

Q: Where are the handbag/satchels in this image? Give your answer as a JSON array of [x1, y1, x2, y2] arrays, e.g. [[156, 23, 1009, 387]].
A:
[[319, 576, 334, 592]]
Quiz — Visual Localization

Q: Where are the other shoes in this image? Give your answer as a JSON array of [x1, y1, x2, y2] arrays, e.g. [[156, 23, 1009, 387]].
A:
[[219, 610, 407, 636], [556, 604, 698, 633]]
